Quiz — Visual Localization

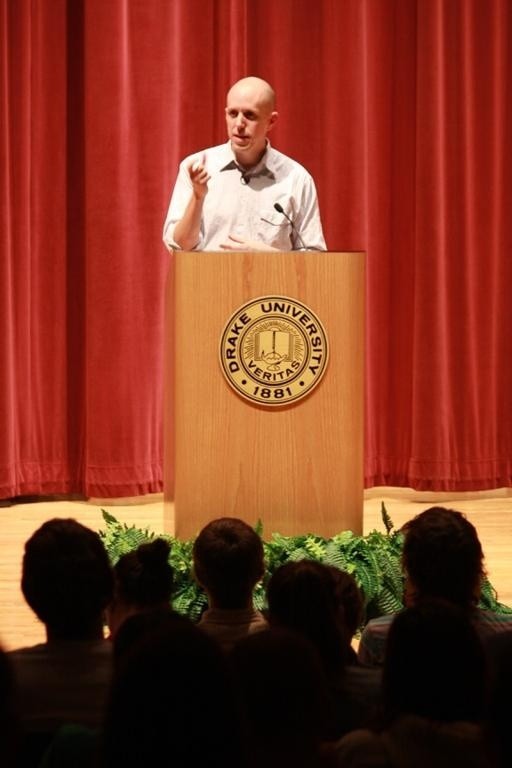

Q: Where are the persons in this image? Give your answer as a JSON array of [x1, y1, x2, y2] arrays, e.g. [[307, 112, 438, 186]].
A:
[[160, 76, 328, 254], [1, 517, 114, 737], [64, 607, 511, 768], [258, 559, 365, 661], [103, 517, 270, 653], [1, 645, 63, 768], [354, 506, 511, 669]]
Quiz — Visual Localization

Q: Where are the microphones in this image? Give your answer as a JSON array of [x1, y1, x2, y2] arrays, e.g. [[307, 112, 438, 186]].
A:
[[274, 203, 309, 251]]
